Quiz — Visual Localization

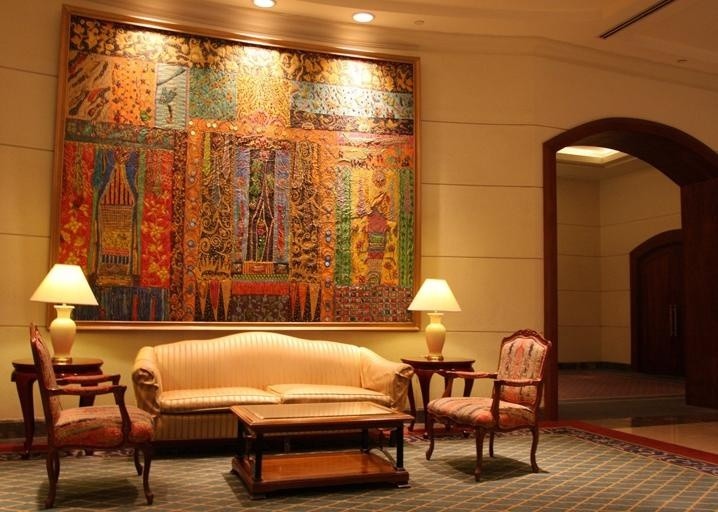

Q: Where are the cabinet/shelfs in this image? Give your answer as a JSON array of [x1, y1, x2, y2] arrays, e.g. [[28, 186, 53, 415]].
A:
[[400, 357, 476, 459]]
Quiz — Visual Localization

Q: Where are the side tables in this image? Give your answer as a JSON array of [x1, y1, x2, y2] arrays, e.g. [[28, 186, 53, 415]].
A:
[[11, 357, 104, 460]]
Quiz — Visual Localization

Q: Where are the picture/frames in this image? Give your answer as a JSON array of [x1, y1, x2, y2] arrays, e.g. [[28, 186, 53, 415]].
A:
[[45, 5, 423, 332]]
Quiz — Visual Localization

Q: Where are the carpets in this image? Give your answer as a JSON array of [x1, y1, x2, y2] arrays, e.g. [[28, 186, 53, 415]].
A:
[[0, 420, 718, 512]]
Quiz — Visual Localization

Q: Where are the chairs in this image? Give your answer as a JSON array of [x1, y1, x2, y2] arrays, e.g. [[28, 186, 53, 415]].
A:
[[25, 321, 158, 502], [423, 328, 553, 481]]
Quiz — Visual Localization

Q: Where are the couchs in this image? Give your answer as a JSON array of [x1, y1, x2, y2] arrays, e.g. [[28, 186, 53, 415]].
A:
[[132, 327, 414, 454]]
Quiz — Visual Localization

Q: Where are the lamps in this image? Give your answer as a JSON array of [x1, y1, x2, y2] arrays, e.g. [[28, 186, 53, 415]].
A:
[[30, 264, 100, 362], [408, 278, 461, 361]]
